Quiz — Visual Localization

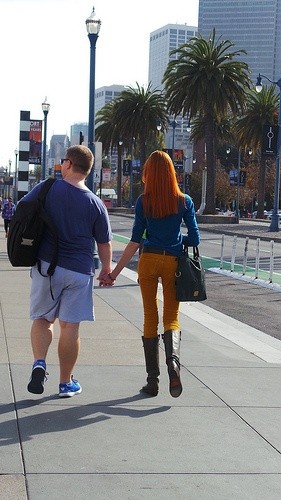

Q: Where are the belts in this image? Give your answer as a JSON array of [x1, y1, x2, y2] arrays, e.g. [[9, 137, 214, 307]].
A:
[[144, 247, 172, 256]]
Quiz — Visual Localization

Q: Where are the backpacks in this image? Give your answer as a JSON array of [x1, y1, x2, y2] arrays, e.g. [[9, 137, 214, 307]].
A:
[[6, 178, 57, 278]]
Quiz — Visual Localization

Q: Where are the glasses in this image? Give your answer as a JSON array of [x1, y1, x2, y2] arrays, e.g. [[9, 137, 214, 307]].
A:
[[61, 158, 72, 164]]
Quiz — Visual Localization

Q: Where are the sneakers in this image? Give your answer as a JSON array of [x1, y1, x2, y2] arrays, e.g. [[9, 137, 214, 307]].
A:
[[27, 360, 49, 394], [58, 375, 82, 397]]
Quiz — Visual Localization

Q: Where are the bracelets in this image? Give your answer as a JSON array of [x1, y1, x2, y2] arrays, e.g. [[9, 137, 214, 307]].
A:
[[109, 275, 116, 280]]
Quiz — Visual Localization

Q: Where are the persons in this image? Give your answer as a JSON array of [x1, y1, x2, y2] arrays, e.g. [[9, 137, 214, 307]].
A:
[[2, 198, 16, 237], [100, 151, 201, 398], [18, 144, 112, 396]]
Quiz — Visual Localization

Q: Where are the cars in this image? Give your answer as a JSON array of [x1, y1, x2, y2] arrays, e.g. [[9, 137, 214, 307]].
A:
[[216, 208, 281, 220], [95, 189, 117, 207]]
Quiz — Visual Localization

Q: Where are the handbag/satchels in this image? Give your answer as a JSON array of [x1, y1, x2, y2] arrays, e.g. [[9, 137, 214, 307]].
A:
[[175, 235, 207, 302]]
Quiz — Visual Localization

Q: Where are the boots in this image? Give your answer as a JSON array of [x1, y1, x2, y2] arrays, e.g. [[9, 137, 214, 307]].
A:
[[141, 334, 160, 395], [161, 329, 182, 398]]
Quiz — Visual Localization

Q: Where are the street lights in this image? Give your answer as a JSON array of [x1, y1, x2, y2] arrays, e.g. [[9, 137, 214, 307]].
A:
[[84, 6, 102, 268], [225, 144, 253, 219], [255, 71, 281, 232], [13, 148, 19, 205], [42, 98, 52, 182], [4, 158, 12, 201], [156, 119, 192, 161], [118, 135, 134, 207]]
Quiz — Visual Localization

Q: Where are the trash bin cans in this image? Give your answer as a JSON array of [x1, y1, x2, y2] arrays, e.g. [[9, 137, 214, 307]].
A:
[[240, 211, 248, 218], [104, 198, 113, 208]]
